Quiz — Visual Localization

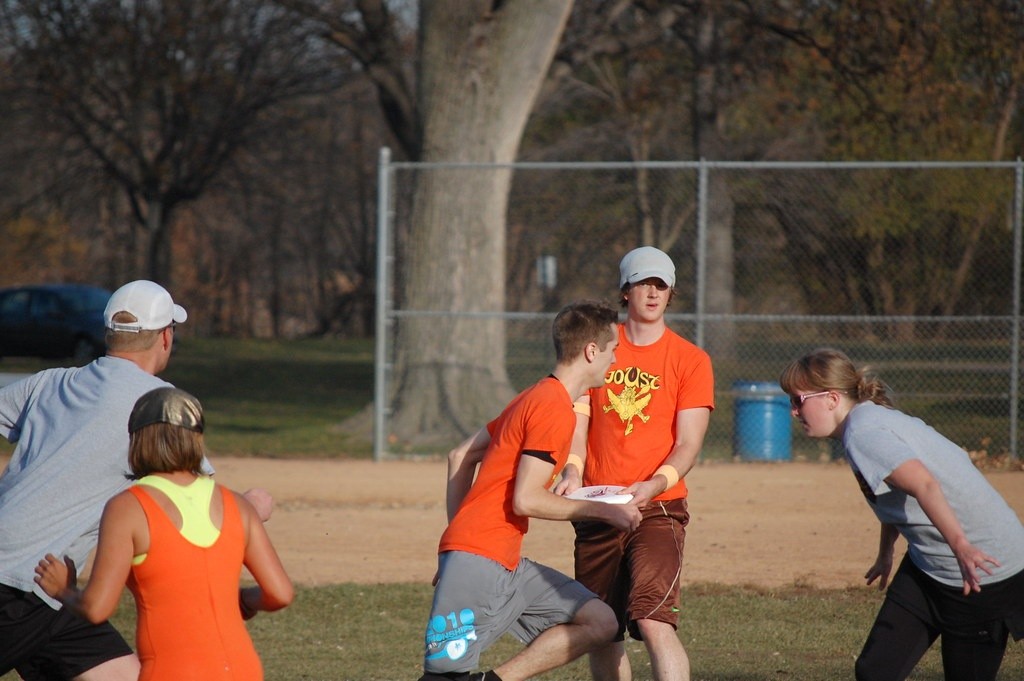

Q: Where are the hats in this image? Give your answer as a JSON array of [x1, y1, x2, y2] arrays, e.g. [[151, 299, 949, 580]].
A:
[[103, 280, 188, 333], [619, 246, 676, 289], [128, 387, 205, 434]]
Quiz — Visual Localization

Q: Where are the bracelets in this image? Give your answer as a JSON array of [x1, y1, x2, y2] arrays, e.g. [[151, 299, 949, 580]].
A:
[[651, 465, 679, 492], [560, 454, 584, 478]]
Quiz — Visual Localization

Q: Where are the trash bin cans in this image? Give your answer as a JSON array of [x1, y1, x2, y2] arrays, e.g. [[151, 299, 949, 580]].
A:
[[731, 379, 794, 463]]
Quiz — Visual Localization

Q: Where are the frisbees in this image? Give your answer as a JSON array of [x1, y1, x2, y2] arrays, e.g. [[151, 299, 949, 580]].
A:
[[565, 484, 635, 506]]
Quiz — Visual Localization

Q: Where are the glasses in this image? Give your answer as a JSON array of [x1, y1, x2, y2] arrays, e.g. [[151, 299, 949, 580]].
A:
[[788, 390, 848, 409], [157, 320, 176, 334]]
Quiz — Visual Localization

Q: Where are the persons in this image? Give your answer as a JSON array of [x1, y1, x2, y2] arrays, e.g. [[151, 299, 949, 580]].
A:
[[780, 347, 1024, 681], [418, 303, 643, 681], [557, 244, 714, 681], [0, 280, 271, 681], [33, 387, 296, 681]]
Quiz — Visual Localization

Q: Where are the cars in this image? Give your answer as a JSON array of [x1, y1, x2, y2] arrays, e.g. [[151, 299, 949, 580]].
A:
[[0, 284, 113, 368]]
